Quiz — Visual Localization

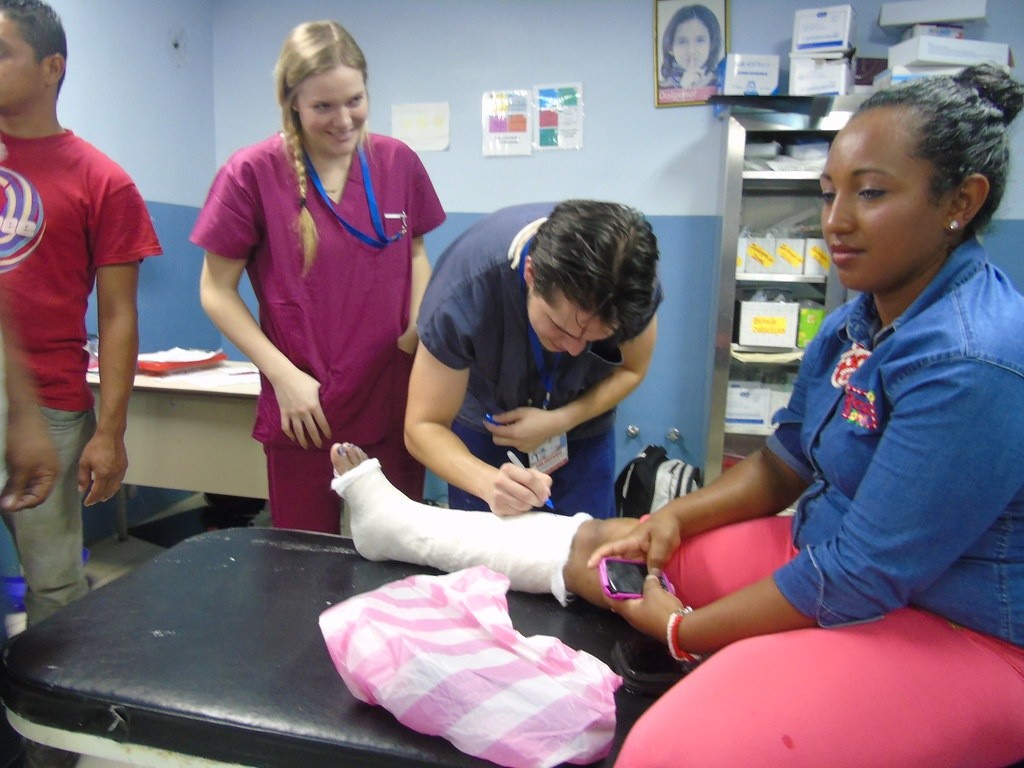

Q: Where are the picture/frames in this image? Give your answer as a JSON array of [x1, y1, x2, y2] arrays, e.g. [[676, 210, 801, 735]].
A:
[[654, 0, 730, 107]]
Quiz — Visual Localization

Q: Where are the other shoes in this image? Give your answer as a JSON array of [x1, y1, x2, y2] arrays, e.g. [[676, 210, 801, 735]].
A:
[[25, 739, 81, 768]]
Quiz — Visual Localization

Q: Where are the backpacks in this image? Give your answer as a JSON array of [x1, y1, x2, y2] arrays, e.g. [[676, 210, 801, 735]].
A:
[[614, 443, 702, 520]]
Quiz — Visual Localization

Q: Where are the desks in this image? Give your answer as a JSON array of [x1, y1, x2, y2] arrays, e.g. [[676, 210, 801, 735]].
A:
[[85, 360, 351, 542]]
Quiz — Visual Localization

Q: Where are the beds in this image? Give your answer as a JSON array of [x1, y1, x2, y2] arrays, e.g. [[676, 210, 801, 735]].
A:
[[0, 526, 1024, 768]]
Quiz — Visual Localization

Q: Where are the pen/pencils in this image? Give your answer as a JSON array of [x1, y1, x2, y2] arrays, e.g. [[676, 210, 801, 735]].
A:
[[506, 450, 556, 511]]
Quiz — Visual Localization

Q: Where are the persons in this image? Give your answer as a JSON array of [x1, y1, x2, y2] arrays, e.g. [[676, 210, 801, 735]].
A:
[[331, 63, 1024, 768], [189, 20, 445, 535], [402, 200, 664, 521], [662, 5, 723, 89], [1, 0, 164, 645]]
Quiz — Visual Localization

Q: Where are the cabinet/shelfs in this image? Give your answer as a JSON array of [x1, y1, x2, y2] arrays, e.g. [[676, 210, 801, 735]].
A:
[[705, 94, 871, 517]]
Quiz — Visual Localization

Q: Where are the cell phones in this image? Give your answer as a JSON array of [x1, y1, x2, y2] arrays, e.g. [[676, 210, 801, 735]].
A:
[[599, 557, 674, 600]]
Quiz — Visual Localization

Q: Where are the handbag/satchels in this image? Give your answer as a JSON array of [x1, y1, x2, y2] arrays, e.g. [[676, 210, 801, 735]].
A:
[[317, 565, 624, 768]]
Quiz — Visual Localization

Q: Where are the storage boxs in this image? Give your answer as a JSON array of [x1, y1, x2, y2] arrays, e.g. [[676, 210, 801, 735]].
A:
[[725, 367, 799, 425], [715, 0, 1015, 96], [745, 140, 829, 159], [735, 237, 833, 276], [736, 297, 824, 348]]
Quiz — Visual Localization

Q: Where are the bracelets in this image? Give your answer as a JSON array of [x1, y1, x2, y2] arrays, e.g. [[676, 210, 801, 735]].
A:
[[666, 606, 707, 665]]
